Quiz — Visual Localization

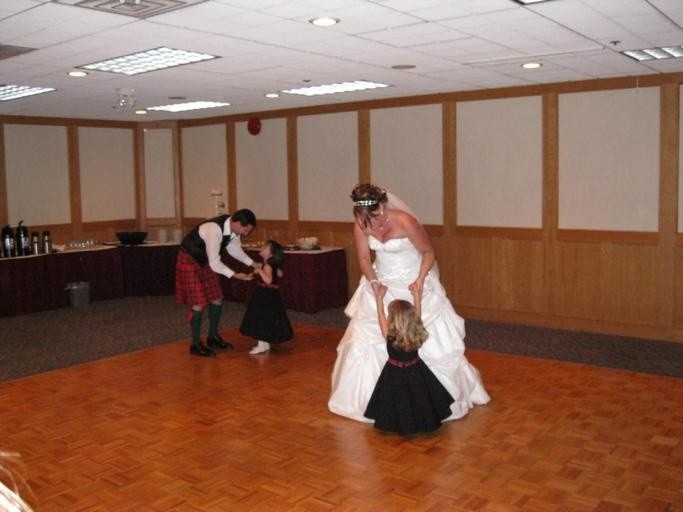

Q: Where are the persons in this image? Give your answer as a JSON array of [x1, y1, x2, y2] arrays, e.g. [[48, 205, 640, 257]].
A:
[[174, 208, 262, 357], [240, 239, 294, 354], [327, 182, 492, 424], [364, 283, 455, 436]]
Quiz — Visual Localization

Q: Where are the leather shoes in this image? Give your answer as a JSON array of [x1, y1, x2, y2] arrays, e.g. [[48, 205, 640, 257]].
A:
[[208, 335, 232, 349], [191, 344, 215, 356]]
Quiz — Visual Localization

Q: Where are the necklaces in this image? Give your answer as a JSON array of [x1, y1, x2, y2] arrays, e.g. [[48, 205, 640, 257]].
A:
[[380, 214, 389, 230]]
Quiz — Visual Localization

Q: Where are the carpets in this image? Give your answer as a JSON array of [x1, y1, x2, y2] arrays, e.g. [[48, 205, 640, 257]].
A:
[[1, 293, 682, 385]]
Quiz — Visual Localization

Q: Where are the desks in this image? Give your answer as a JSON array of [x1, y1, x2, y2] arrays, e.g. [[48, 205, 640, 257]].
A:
[[1, 241, 348, 317]]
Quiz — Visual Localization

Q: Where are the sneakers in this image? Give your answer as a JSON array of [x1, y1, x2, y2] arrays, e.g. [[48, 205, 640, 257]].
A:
[[248, 342, 271, 354]]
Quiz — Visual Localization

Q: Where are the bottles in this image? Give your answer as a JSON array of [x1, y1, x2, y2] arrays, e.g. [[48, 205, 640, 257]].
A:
[[2, 221, 52, 256]]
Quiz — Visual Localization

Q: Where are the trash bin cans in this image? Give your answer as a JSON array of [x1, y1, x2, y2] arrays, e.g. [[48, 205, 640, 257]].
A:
[[69, 282, 88, 308]]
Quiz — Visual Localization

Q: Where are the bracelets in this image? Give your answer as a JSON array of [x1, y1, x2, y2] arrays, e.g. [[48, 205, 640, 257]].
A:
[[369, 280, 377, 283]]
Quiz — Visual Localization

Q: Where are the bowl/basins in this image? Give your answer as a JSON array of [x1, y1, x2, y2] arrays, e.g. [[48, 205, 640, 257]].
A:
[[116, 232, 147, 244], [295, 239, 319, 250]]
